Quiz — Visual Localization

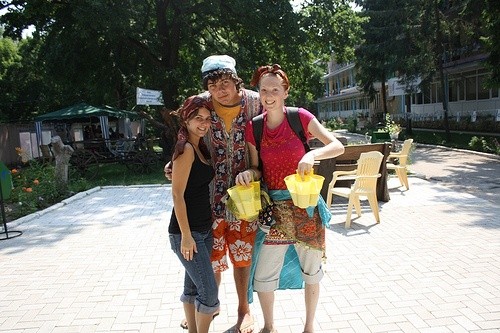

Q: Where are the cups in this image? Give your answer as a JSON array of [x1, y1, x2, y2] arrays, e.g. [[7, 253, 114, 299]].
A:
[[283, 173, 325, 209], [225, 181, 264, 223]]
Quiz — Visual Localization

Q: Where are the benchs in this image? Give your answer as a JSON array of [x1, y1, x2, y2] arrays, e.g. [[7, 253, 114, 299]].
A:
[[309, 142, 391, 202]]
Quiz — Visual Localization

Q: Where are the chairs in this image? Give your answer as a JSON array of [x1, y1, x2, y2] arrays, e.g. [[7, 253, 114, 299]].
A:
[[386, 139, 413, 190], [327, 151, 384, 229]]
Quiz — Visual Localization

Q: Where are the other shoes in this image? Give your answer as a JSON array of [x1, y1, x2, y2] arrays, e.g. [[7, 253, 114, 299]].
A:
[[224, 320, 256, 333], [180, 308, 220, 328]]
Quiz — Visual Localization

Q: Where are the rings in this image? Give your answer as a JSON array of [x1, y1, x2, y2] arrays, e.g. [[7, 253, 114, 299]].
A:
[[301, 166, 304, 171], [186, 251, 189, 253]]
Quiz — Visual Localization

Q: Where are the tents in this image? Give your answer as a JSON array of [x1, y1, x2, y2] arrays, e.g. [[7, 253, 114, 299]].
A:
[[34, 101, 151, 138]]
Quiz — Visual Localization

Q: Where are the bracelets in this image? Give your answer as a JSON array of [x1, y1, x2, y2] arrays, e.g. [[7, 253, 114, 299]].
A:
[[248, 168, 257, 181]]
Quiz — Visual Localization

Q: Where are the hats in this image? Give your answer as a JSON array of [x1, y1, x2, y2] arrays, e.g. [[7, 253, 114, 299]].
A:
[[201, 55, 237, 74]]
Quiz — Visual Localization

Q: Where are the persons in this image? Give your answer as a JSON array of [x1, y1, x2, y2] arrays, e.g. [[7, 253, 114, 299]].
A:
[[321, 120, 327, 127], [48, 124, 149, 157], [167, 96, 221, 333], [164, 55, 266, 333], [235, 64, 346, 333]]
